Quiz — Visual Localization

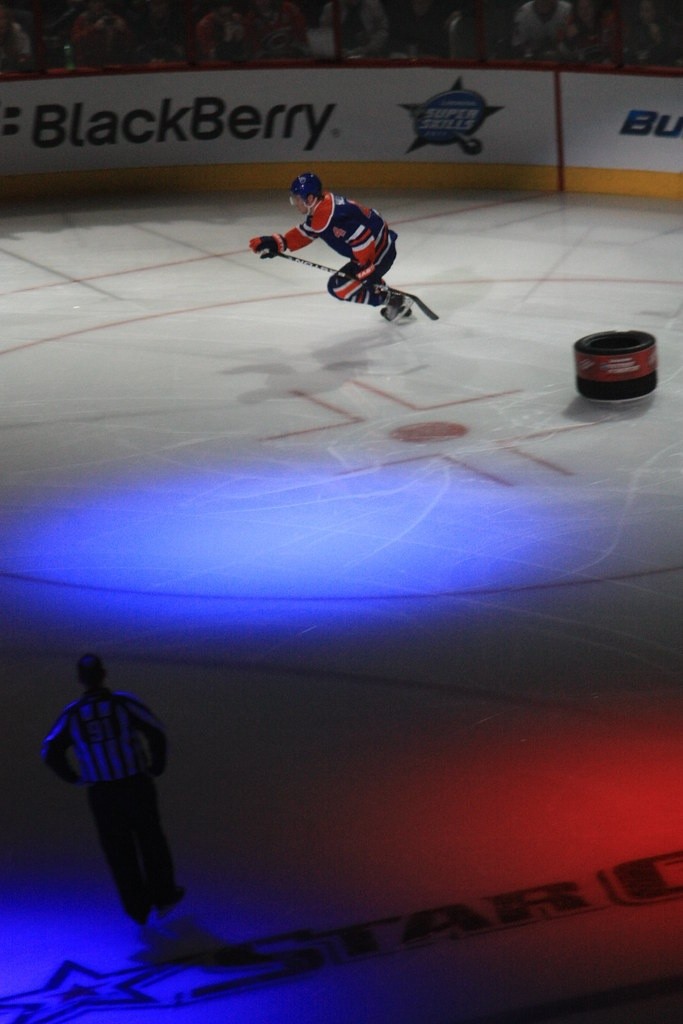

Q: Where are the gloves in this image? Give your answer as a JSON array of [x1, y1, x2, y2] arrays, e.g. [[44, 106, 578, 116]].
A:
[[249, 234, 286, 259], [352, 260, 378, 286]]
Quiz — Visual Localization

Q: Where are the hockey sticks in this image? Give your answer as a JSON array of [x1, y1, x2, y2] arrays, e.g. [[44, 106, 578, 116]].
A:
[[259, 247, 441, 322]]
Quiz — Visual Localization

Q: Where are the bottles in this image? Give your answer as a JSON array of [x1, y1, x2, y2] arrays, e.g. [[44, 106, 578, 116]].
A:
[[63, 42, 76, 70]]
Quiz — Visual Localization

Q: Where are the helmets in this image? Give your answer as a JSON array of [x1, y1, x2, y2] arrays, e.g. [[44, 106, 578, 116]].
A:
[[291, 173, 321, 201]]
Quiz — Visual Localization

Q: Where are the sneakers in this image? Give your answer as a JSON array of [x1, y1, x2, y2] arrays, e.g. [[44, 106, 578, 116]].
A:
[[381, 291, 412, 322], [397, 309, 417, 324]]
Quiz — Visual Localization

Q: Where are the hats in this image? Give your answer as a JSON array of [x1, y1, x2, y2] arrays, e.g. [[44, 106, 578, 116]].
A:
[[78, 654, 105, 681]]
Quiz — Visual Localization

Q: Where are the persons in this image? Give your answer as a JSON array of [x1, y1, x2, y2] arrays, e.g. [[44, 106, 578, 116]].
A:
[[249, 172, 411, 321], [0, 0, 683, 72], [41, 653, 186, 925]]
